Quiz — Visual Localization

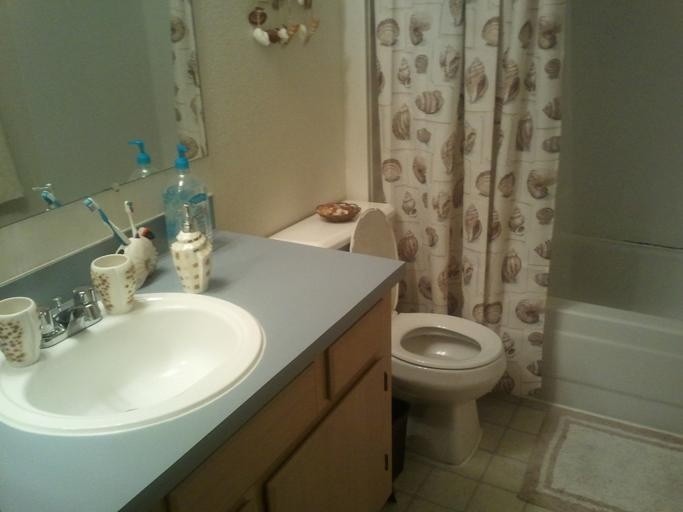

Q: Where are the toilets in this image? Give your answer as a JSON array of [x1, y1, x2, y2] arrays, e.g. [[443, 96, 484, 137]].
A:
[[263, 200, 508, 465]]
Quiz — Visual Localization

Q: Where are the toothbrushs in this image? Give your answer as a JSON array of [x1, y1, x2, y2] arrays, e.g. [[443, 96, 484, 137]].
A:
[[124, 200, 140, 239], [83, 197, 131, 246], [41, 190, 62, 207]]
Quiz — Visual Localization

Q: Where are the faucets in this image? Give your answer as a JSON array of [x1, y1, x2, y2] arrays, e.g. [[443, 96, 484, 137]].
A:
[[38, 285, 104, 350]]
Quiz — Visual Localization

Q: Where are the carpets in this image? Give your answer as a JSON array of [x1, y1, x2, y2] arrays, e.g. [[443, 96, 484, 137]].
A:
[[515, 403, 683, 512]]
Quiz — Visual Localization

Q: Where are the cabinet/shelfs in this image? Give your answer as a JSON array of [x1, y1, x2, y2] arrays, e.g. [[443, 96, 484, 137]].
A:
[[118, 282, 397, 512]]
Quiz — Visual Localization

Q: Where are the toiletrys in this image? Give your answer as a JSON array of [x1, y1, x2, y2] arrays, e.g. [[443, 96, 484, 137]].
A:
[[170, 202, 213, 293], [31, 183, 56, 211]]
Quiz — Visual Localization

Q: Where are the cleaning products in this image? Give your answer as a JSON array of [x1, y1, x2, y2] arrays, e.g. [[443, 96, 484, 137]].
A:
[[127, 139, 160, 182], [160, 143, 211, 248]]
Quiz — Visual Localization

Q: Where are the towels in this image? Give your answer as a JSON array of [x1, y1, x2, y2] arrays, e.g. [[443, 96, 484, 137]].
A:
[[0, 123, 27, 207]]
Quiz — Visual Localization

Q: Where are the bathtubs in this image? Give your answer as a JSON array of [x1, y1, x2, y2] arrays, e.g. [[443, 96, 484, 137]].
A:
[[524, 233, 682, 436]]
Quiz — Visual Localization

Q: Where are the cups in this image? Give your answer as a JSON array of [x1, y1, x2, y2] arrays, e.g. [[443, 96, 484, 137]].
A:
[[88, 253, 137, 317], [0, 296, 43, 369]]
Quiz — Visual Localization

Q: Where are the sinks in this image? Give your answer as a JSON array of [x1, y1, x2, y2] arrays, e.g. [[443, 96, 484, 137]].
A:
[[0, 292, 267, 438]]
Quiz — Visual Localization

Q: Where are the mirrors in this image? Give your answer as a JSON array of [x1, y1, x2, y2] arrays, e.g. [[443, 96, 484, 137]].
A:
[[0, 0, 209, 232]]
